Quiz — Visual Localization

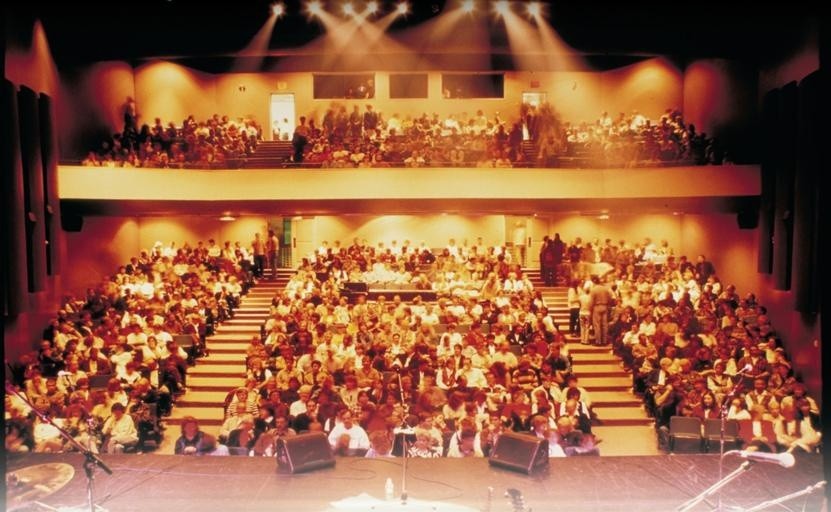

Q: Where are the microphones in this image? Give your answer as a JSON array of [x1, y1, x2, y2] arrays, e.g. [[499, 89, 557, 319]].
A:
[[392, 364, 400, 372], [730, 450, 795, 469], [735, 364, 752, 377]]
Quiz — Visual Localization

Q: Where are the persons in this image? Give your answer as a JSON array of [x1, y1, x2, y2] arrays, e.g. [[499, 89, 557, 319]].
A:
[[79, 76, 734, 168], [4, 219, 824, 455]]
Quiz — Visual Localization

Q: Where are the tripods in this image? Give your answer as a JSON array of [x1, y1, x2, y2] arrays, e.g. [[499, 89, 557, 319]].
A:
[[370, 372, 437, 511]]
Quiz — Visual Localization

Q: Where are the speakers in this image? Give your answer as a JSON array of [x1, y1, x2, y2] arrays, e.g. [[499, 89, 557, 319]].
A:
[[488, 432, 548, 474], [276, 431, 336, 475]]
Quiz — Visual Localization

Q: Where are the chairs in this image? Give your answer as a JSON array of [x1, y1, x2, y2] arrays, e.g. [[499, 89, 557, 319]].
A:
[[433, 322, 521, 355], [671, 417, 733, 458], [369, 282, 438, 302], [174, 334, 194, 354]]
